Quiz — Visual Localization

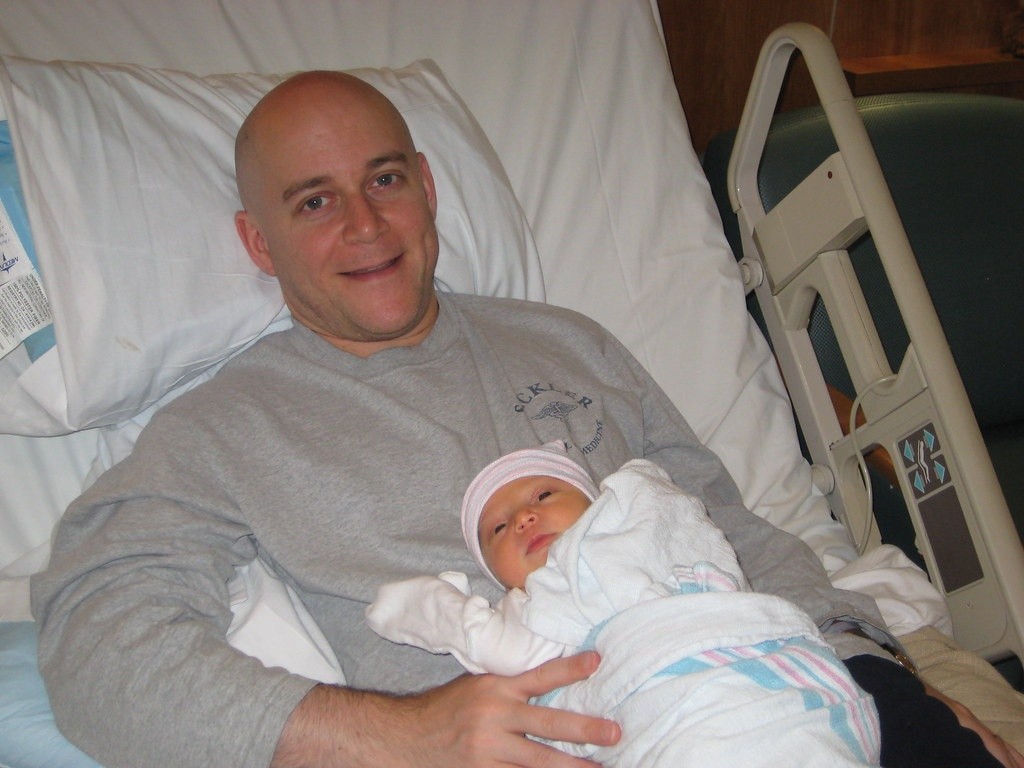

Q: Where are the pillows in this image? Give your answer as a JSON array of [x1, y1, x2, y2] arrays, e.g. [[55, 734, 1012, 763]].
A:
[[0, 57, 545, 438]]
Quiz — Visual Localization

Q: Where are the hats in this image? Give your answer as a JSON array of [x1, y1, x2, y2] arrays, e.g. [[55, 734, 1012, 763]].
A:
[[460, 438, 602, 593]]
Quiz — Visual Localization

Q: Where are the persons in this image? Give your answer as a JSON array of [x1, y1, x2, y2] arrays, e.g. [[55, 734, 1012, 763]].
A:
[[366, 440, 880, 767], [32, 70, 1024, 768]]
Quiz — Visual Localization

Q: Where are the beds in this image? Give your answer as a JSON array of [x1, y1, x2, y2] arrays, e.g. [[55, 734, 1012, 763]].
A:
[[1, 0, 1024, 767]]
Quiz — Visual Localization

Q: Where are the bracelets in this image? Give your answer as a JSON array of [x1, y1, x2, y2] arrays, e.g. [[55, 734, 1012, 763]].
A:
[[881, 643, 918, 675]]
